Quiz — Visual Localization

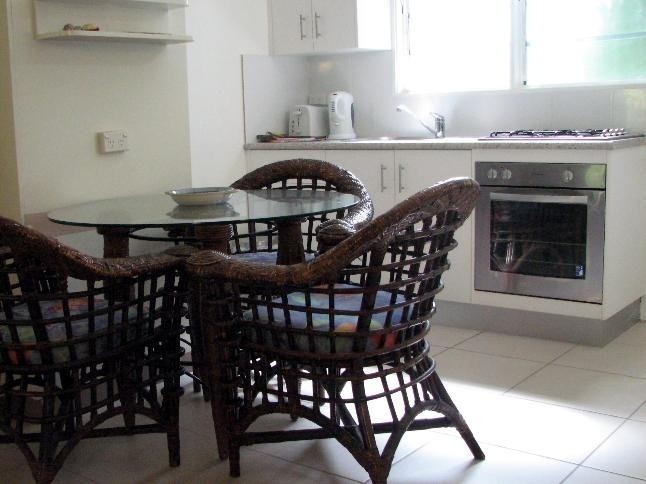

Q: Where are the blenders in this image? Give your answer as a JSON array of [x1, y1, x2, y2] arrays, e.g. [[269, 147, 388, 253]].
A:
[[327, 92, 356, 142]]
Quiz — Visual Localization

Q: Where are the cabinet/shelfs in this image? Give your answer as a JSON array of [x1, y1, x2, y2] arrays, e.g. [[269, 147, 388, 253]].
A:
[[268, 0, 392, 57], [245, 149, 471, 305], [31, 0, 195, 45], [97, 129, 129, 154]]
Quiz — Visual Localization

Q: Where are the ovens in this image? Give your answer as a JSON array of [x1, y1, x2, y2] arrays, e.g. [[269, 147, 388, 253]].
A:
[[471, 162, 608, 306]]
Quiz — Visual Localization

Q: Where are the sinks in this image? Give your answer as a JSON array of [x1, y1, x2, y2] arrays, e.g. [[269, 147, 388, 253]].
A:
[[388, 137, 434, 141]]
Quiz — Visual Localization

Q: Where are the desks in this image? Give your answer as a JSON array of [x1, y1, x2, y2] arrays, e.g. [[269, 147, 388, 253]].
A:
[[45, 185, 361, 278]]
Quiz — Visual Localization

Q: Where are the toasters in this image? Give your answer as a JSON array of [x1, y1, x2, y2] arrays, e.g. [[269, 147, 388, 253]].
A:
[[288, 104, 329, 138]]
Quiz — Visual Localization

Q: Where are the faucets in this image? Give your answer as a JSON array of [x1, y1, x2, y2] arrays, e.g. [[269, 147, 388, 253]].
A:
[[397, 104, 445, 138]]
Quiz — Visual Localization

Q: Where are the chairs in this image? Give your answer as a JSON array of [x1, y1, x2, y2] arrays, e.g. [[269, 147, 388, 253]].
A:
[[208, 157, 375, 285], [0, 214, 194, 482], [193, 176, 486, 483]]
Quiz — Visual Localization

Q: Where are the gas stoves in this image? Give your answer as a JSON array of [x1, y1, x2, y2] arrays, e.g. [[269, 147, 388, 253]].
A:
[[479, 127, 644, 142]]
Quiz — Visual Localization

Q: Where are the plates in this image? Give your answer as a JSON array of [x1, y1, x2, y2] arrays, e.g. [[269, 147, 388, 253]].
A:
[[164, 187, 237, 206]]
